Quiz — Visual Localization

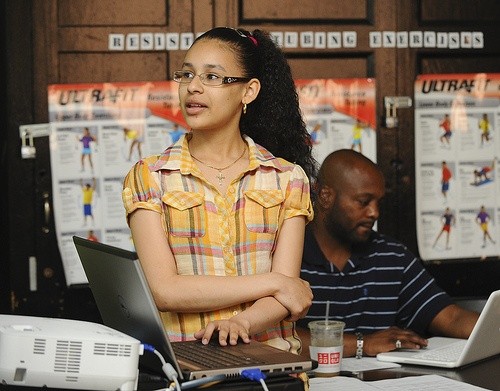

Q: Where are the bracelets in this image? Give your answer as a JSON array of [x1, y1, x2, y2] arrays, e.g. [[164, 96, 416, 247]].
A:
[[356, 336, 364, 357]]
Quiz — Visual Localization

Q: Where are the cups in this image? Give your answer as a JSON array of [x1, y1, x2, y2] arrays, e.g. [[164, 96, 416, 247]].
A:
[[308, 320, 345, 377]]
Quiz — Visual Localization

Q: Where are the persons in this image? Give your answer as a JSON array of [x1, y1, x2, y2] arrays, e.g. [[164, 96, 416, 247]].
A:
[[122, 28, 311, 391], [296, 149, 480, 360], [77, 113, 495, 252]]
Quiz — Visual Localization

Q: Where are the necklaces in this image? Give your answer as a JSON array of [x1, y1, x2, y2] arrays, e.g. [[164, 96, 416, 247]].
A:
[[189, 144, 247, 187]]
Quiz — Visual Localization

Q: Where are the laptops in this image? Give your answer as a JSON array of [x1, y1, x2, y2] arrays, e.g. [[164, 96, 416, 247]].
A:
[[72, 236, 318, 384], [377, 291, 500, 368]]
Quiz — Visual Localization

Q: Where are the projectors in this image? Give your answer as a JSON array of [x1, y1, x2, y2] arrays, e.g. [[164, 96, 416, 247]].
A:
[[0, 315, 140, 391]]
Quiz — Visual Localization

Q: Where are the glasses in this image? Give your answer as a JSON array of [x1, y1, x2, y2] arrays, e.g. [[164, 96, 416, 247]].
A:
[[172, 70, 250, 86]]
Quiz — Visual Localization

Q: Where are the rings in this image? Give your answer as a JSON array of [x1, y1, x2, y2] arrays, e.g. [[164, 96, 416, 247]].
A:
[[395, 339, 402, 349]]
